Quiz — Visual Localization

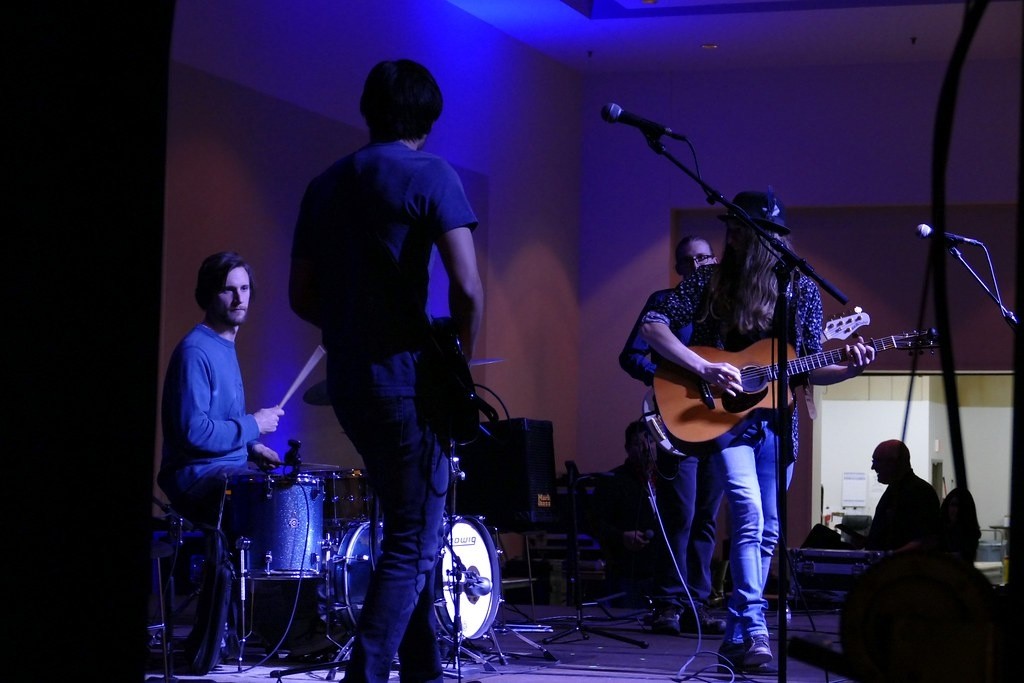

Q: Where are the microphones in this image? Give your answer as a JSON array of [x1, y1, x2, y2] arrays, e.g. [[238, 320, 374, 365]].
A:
[[464, 574, 493, 597], [914, 224, 984, 248], [601, 104, 688, 143]]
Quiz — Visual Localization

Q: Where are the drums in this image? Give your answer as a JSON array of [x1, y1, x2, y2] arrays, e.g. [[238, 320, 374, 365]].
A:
[[301, 467, 382, 526], [226, 474, 325, 580], [331, 514, 502, 643]]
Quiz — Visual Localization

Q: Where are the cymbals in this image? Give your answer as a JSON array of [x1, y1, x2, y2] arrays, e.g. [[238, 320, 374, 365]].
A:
[[303, 380, 332, 406], [468, 358, 510, 366]]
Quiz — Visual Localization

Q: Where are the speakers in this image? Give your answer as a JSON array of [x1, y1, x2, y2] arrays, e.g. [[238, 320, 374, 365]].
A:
[[449, 419, 560, 532]]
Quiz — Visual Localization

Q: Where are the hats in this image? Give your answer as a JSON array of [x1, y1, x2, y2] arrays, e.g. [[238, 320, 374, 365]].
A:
[[717, 190, 792, 235]]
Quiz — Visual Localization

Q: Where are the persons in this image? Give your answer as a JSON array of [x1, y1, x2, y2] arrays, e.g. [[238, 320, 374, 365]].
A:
[[288, 59, 483, 683], [835, 440, 942, 673], [157, 251, 345, 653], [599, 421, 671, 611], [615, 233, 727, 636], [641, 190, 876, 674]]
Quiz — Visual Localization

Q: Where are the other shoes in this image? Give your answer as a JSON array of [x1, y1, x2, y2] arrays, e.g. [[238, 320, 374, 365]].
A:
[[743, 635, 773, 664], [717, 646, 779, 675], [680, 607, 727, 635], [653, 610, 680, 633]]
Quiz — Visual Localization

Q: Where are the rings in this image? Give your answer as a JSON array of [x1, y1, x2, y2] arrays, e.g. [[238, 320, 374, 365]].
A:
[[848, 355, 855, 363]]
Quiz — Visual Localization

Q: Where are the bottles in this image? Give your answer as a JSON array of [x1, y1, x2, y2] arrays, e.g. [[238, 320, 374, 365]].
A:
[[777, 601, 791, 626]]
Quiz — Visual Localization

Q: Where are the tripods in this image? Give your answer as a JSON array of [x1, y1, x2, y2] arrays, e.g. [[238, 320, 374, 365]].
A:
[[541, 463, 650, 649]]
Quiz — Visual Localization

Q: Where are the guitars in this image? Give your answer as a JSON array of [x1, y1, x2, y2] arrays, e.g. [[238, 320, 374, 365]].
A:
[[643, 306, 870, 456], [653, 327, 941, 456]]
